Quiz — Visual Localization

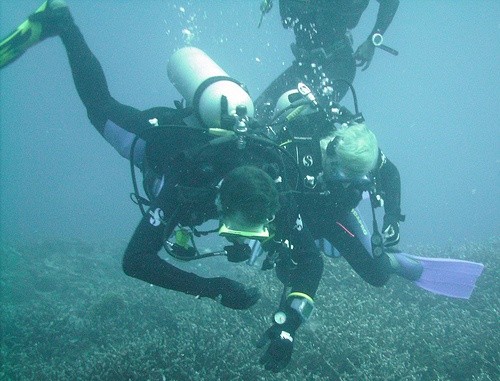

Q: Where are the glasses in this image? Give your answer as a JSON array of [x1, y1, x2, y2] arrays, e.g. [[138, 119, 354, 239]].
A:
[[332, 169, 376, 186], [218, 223, 269, 240]]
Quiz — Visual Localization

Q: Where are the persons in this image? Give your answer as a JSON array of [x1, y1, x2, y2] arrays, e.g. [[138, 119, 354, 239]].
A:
[[253, 0, 400, 128], [0, 0, 325, 372], [257, 89, 485, 300]]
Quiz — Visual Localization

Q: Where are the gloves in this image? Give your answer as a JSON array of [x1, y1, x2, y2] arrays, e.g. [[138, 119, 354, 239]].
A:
[[188, 273, 262, 310], [381, 214, 401, 248], [280, 239, 298, 272], [256, 310, 302, 371]]
